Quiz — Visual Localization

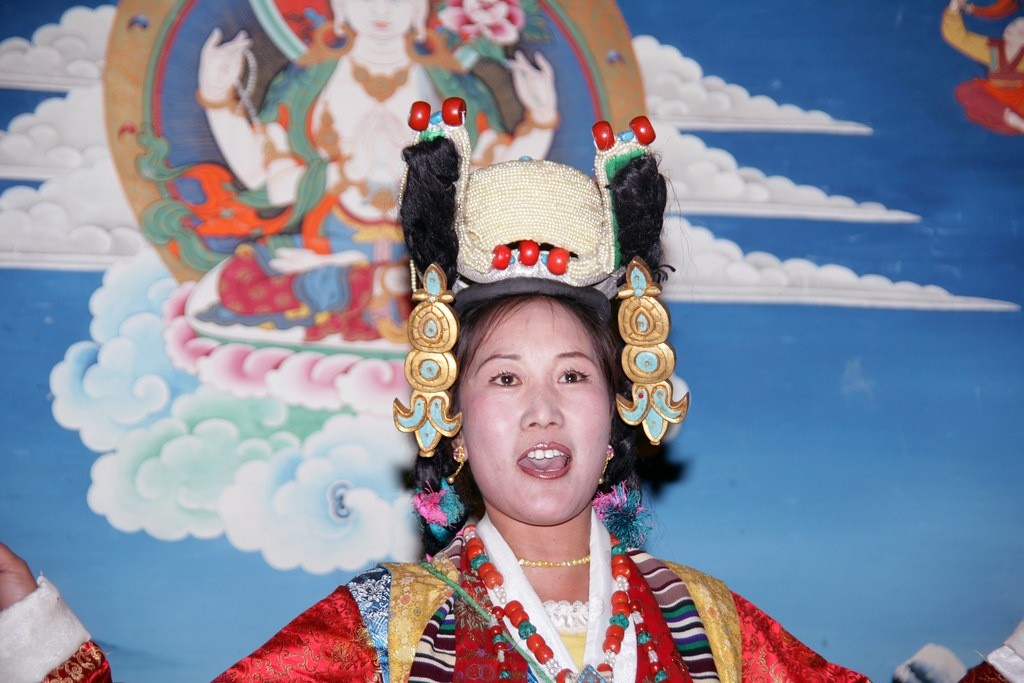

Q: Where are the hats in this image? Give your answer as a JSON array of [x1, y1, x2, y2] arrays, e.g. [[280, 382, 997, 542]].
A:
[[392, 96, 692, 547]]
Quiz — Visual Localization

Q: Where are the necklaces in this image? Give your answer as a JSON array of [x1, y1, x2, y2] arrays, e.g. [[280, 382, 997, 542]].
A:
[[418, 524, 670, 683]]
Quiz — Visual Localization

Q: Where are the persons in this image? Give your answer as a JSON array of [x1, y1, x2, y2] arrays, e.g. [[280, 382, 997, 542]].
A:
[[0, 136, 1024, 683]]
